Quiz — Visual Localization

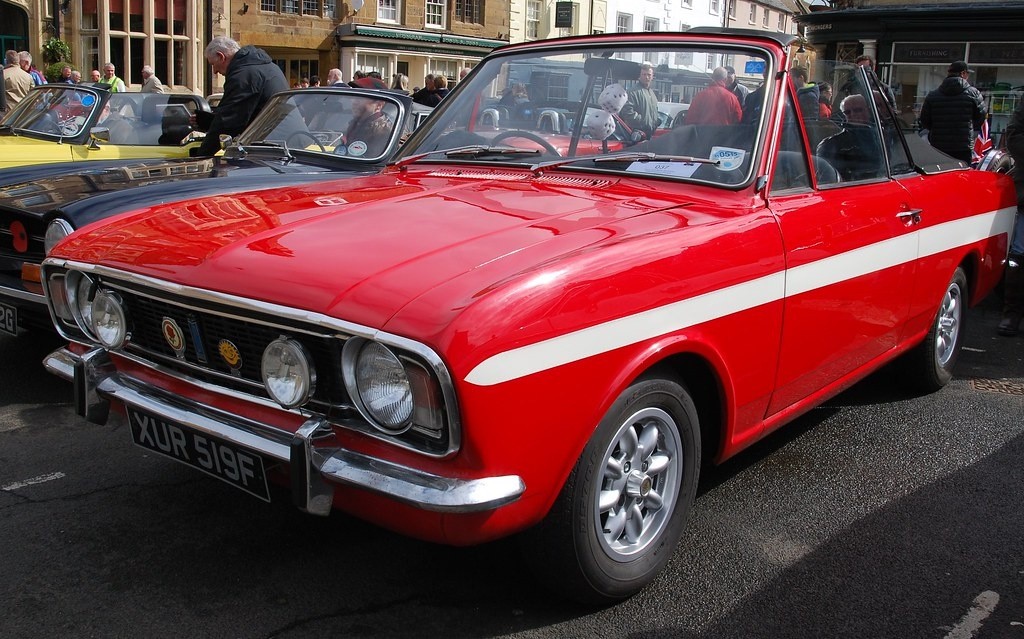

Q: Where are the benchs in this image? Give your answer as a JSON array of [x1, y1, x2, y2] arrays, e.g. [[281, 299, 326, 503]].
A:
[[647, 122, 901, 177]]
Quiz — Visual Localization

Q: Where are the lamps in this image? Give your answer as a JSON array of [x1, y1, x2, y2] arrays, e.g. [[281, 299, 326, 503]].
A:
[[795, 43, 806, 53]]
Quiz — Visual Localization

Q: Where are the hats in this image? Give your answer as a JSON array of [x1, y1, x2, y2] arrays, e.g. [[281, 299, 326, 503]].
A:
[[349, 77, 389, 92], [947, 60, 975, 74]]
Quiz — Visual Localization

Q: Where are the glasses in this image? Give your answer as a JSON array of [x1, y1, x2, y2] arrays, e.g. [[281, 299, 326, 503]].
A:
[[843, 106, 868, 115]]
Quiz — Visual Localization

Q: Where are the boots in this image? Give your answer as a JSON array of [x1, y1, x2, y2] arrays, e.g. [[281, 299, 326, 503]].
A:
[[997, 252, 1024, 336]]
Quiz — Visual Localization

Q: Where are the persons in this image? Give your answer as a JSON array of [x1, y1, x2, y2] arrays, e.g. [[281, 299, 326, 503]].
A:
[[140, 65, 164, 92], [498, 82, 527, 106], [789, 64, 820, 120], [724, 66, 749, 107], [815, 95, 892, 181], [60, 62, 126, 92], [0, 50, 51, 119], [742, 64, 792, 124], [292, 69, 392, 156], [76, 94, 139, 143], [817, 81, 834, 118], [186, 36, 311, 159], [920, 60, 986, 165], [831, 55, 897, 122], [1005, 91, 1024, 263], [685, 66, 743, 126], [391, 66, 481, 132], [620, 65, 657, 138], [872, 91, 909, 152]]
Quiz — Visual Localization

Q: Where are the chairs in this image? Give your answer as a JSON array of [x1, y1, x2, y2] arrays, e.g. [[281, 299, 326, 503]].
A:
[[715, 152, 843, 190]]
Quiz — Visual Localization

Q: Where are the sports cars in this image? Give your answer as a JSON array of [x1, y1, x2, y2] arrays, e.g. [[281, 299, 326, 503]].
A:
[[0, 87, 673, 334], [0, 83, 336, 170], [43, 27, 1017, 600]]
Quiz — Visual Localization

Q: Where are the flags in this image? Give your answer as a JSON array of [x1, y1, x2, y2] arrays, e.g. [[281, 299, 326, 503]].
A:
[[971, 117, 992, 169]]
